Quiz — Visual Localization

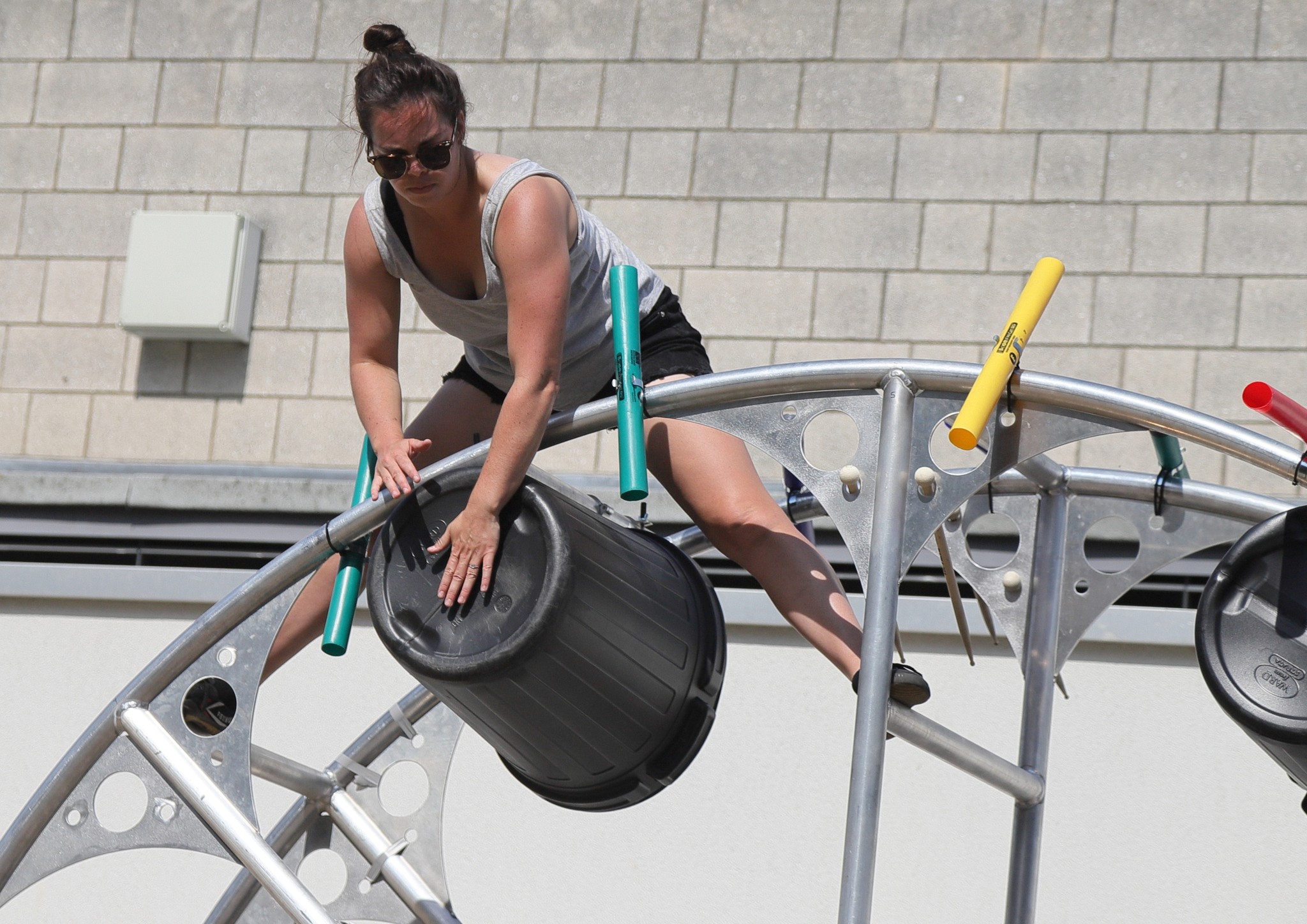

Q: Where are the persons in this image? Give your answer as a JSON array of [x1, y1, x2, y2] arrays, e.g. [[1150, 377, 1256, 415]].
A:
[[184, 22, 930, 742]]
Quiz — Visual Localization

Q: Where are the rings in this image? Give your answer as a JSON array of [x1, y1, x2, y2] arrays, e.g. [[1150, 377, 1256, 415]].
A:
[[468, 564, 480, 569]]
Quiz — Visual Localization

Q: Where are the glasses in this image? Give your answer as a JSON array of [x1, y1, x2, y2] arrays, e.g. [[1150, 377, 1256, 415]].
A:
[[367, 116, 459, 180]]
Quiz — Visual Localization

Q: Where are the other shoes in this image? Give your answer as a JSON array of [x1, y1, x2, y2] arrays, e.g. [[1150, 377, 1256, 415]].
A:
[[181, 677, 236, 735], [885, 663, 932, 742]]
